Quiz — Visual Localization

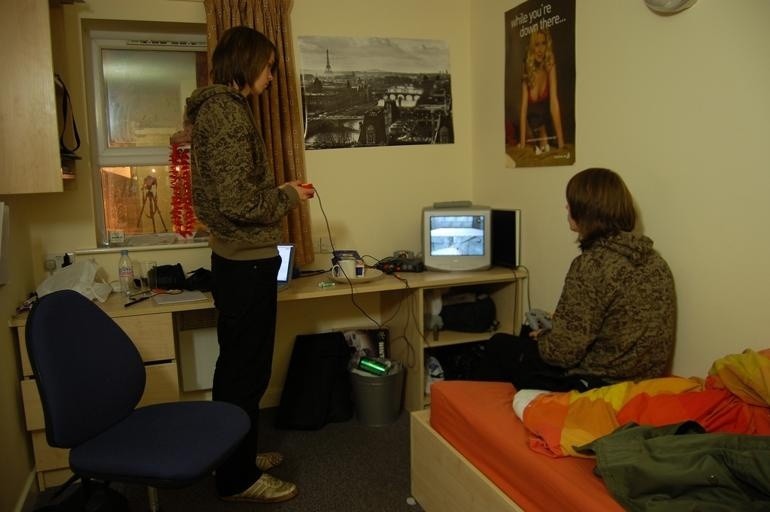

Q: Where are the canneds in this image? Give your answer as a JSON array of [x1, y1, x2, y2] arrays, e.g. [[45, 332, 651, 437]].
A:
[[360, 357, 389, 375]]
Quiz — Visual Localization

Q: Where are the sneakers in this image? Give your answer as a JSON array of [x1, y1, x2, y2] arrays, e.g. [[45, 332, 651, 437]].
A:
[[220, 452, 298, 503]]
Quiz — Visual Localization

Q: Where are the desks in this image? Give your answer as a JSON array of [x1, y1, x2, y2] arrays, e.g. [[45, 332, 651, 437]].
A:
[[8, 264, 529, 493]]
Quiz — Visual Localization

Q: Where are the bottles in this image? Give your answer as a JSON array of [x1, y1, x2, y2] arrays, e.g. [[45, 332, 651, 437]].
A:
[[118, 249, 135, 299]]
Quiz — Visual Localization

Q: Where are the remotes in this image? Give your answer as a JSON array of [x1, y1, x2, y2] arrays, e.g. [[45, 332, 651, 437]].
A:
[[432, 200, 474, 209]]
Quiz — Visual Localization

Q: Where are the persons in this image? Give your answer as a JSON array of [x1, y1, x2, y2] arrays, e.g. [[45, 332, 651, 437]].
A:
[[471, 167, 678, 395], [511, 21, 571, 154], [183, 25, 316, 501]]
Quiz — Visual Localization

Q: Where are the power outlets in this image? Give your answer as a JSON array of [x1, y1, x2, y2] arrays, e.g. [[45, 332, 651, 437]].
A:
[[45, 246, 75, 276]]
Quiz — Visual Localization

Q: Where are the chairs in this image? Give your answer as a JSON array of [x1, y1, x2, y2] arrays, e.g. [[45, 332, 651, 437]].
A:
[[25, 287, 251, 510]]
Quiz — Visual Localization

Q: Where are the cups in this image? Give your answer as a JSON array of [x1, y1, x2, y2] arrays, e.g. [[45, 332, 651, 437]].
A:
[[331, 260, 356, 280], [139, 260, 158, 292]]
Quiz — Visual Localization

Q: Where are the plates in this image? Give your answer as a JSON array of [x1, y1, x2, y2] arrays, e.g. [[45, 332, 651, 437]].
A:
[[329, 268, 384, 284]]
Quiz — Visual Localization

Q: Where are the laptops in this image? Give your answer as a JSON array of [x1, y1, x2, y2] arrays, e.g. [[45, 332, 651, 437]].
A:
[[276, 243, 296, 293]]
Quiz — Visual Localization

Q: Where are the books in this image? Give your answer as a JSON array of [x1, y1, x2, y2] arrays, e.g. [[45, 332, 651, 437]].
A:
[[150, 288, 209, 305]]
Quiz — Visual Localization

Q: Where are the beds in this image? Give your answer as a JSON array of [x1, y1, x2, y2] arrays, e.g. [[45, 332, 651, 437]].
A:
[[409, 348, 770, 512]]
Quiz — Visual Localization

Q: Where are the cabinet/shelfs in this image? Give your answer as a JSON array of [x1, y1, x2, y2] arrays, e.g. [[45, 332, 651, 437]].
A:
[[0, 1, 76, 196]]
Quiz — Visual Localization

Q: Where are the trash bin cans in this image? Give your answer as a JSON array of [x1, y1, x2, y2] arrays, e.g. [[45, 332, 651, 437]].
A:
[[350, 358, 404, 427]]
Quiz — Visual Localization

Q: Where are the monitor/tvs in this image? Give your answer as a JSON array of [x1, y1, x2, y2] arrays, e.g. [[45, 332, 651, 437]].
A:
[[420, 205, 493, 272]]
